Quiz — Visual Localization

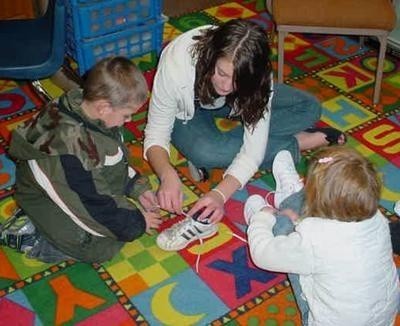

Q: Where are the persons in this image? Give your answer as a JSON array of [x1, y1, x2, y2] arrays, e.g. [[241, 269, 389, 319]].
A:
[[242, 148, 398, 325], [1, 57, 161, 264], [144, 17, 347, 223]]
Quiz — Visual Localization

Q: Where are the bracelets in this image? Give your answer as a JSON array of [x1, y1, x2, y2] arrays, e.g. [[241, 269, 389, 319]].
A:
[[212, 188, 227, 203]]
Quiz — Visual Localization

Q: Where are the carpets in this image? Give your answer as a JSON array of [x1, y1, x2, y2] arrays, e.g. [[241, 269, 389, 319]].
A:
[[0, 0, 399, 325]]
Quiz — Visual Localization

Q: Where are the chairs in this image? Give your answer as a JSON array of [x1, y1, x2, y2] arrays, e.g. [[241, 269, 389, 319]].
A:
[[267, 0, 396, 106]]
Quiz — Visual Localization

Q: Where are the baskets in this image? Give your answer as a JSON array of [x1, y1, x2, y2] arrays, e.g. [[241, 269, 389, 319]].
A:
[[65, 0, 165, 80]]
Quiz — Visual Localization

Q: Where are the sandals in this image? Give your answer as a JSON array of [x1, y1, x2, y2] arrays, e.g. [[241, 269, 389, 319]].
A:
[[188, 161, 208, 182], [304, 127, 347, 147]]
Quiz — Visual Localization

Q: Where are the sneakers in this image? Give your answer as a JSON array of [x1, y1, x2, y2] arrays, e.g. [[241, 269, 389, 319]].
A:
[[156, 218, 217, 251], [0, 209, 37, 253], [243, 194, 270, 226], [272, 150, 304, 209]]
